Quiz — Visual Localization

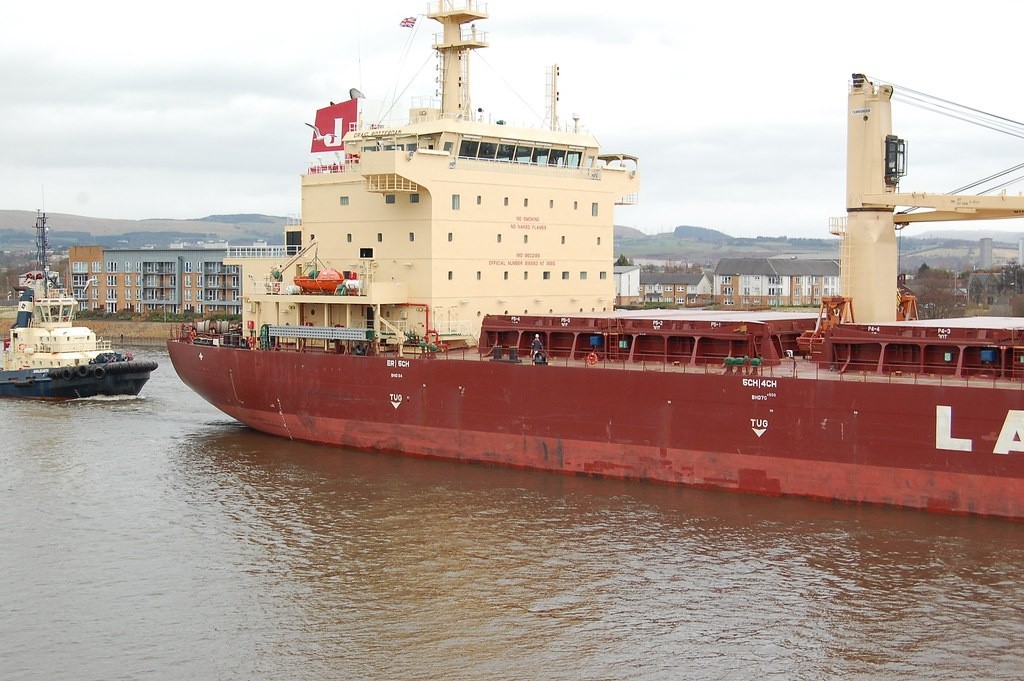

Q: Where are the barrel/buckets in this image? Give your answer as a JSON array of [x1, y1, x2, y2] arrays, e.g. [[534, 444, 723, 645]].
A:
[[223, 333, 230, 344], [229, 329, 237, 334], [493, 346, 502, 359], [509, 346, 518, 360], [232, 335, 239, 345]]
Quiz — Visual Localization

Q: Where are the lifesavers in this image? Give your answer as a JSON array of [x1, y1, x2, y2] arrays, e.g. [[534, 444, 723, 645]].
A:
[[93, 366, 105, 379], [77, 364, 89, 377], [62, 368, 72, 381], [354, 344, 365, 356], [587, 352, 597, 365], [239, 338, 248, 348]]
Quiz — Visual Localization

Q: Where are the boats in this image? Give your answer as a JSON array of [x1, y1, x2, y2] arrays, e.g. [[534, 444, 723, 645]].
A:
[[166, 0, 1024, 523], [0, 209, 158, 401]]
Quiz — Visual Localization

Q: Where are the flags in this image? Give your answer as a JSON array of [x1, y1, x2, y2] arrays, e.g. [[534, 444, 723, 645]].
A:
[[400, 17, 417, 27]]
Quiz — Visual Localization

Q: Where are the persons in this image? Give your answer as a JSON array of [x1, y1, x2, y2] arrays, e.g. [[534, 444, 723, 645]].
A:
[[120, 334, 123, 343], [356, 345, 364, 355], [188, 328, 196, 340], [530, 334, 543, 359]]
